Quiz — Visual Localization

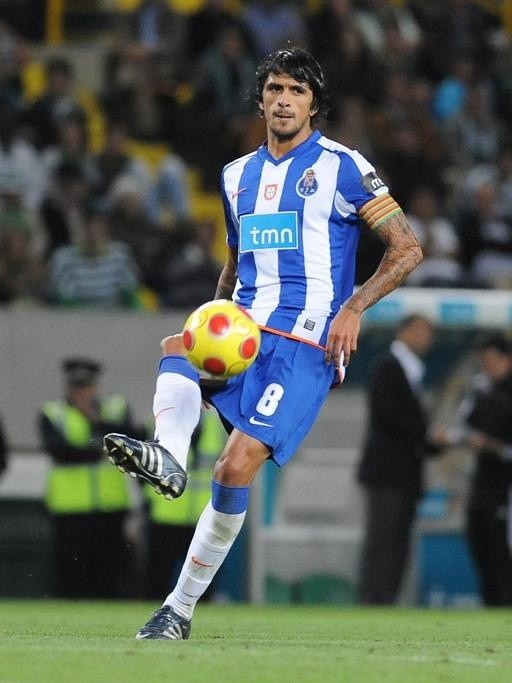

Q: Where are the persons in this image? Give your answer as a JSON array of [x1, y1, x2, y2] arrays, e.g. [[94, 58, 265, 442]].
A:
[[135, 388, 233, 601], [40, 356, 132, 598], [101, 47, 424, 640], [1, 2, 511, 309], [440, 335, 512, 608], [352, 313, 450, 606]]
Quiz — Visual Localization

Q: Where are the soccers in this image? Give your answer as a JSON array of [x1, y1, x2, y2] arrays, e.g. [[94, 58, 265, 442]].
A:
[[181, 299, 261, 377]]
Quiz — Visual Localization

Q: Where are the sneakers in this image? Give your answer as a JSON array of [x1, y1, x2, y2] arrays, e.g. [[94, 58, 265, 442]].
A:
[[103, 433, 188, 501], [135, 605, 192, 641]]
